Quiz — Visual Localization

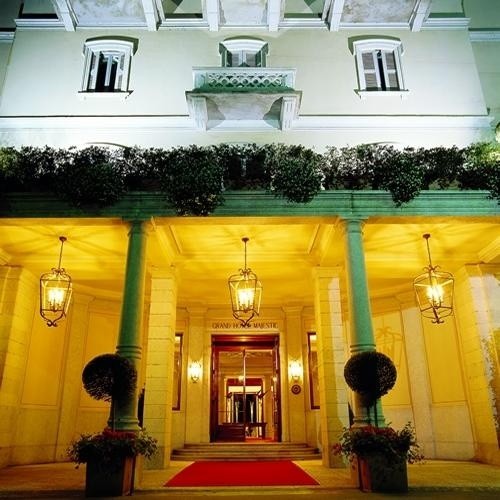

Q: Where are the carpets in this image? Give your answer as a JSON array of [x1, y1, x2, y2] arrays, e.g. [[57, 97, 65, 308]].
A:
[[163, 461, 321, 486]]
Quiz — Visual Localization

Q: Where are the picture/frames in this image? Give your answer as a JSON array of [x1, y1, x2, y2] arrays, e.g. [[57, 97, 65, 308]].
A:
[[307, 331, 320, 409], [172, 332, 183, 410]]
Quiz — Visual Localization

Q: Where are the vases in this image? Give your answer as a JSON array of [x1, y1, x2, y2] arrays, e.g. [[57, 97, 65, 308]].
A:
[[86, 459, 136, 496], [360, 457, 408, 494]]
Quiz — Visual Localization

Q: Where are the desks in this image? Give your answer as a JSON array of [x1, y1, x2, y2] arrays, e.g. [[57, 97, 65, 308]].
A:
[[222, 422, 267, 439]]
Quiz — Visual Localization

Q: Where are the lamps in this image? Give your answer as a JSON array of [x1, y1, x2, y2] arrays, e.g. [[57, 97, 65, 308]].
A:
[[413, 234, 454, 324], [190, 360, 201, 383], [289, 360, 301, 382], [228, 237, 262, 327], [39, 237, 73, 326]]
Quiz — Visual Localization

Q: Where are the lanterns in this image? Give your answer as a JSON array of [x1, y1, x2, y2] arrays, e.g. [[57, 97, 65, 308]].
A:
[[40, 267, 74, 328], [412, 265, 455, 324], [228, 268, 264, 328]]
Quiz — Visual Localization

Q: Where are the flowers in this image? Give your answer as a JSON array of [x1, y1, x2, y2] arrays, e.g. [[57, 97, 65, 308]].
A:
[[65, 353, 157, 470], [332, 352, 426, 471]]
